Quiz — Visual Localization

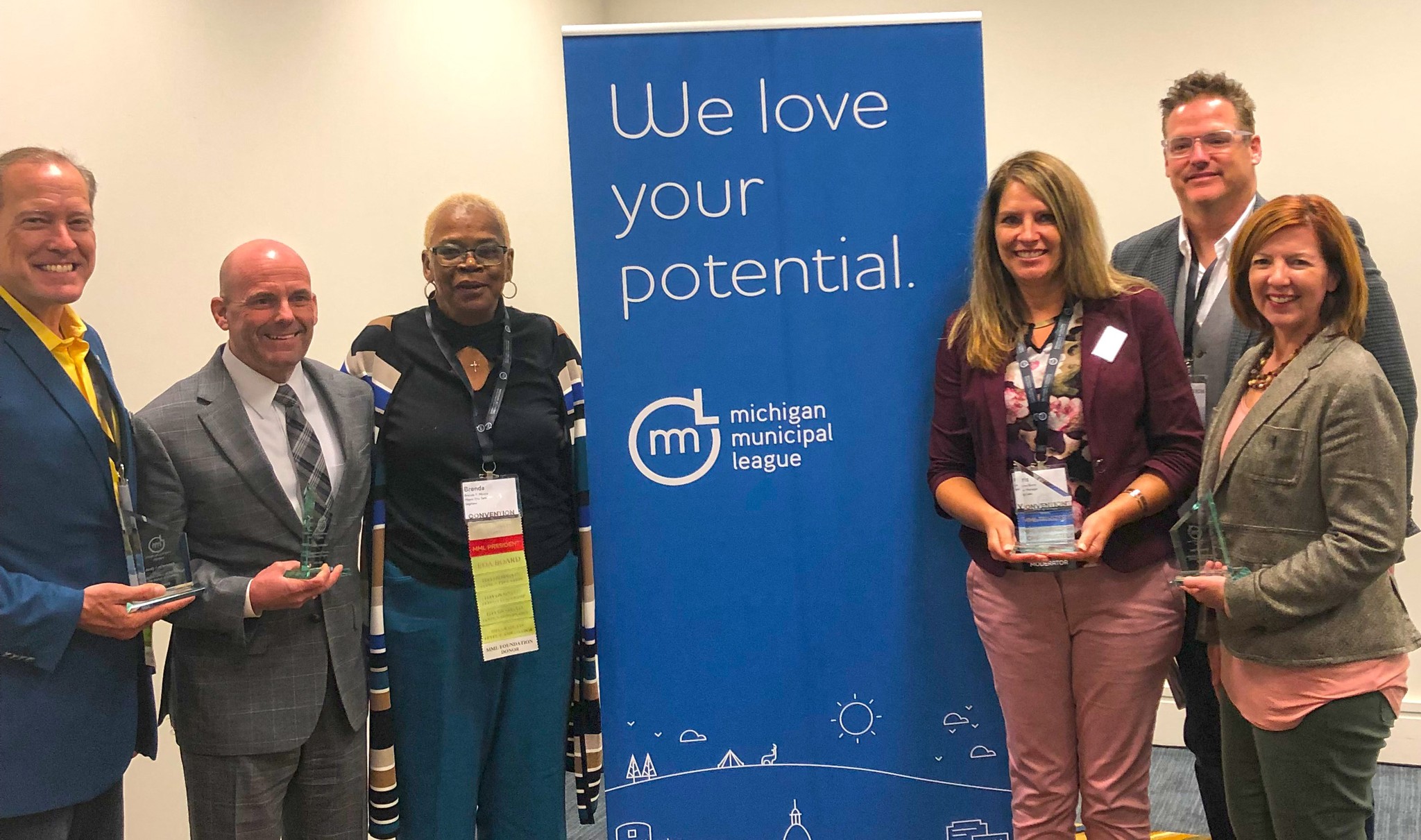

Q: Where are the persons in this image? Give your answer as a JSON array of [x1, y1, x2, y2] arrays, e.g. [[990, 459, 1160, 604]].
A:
[[0, 148, 196, 840], [340, 195, 598, 840], [926, 153, 1205, 840], [132, 240, 376, 840], [1173, 194, 1421, 840], [1107, 73, 1418, 840]]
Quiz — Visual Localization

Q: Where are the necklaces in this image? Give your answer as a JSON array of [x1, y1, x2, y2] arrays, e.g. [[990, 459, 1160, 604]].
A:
[[1248, 321, 1327, 390]]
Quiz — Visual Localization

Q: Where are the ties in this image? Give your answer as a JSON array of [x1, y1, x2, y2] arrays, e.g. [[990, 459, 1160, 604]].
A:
[[274, 383, 334, 540]]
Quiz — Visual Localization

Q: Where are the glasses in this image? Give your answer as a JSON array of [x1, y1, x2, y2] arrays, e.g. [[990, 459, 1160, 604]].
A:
[[429, 243, 508, 268], [1160, 129, 1251, 160]]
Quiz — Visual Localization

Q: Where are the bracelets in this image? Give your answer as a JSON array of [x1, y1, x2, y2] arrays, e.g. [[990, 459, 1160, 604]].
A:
[[1120, 489, 1147, 521]]
